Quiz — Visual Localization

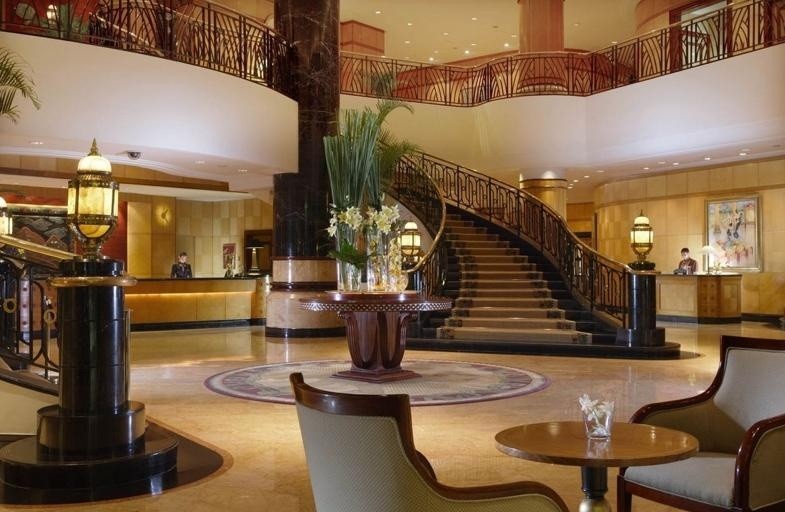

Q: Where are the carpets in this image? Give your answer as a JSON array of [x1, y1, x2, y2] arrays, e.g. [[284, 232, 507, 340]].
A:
[[204, 357, 550, 406]]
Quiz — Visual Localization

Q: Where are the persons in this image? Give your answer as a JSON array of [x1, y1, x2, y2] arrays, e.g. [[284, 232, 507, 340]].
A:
[[170, 251, 192, 279], [679, 247, 697, 275]]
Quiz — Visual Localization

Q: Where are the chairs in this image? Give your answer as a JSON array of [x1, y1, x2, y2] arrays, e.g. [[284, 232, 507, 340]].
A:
[[291, 373, 569, 511], [617, 336, 784, 512]]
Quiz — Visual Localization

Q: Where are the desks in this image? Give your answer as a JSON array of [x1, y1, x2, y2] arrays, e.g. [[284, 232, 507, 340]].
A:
[[495, 420, 700, 512], [302, 292, 452, 384]]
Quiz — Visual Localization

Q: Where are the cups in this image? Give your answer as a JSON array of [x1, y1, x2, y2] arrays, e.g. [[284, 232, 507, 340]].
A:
[[582, 404, 614, 439]]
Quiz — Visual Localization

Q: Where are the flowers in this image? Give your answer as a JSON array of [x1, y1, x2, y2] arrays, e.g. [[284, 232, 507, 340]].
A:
[[322, 93, 420, 258], [580, 392, 614, 425]]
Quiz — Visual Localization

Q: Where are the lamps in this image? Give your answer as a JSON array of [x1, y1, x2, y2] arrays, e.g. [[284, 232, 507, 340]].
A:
[[59, 136, 126, 277], [626, 211, 657, 271]]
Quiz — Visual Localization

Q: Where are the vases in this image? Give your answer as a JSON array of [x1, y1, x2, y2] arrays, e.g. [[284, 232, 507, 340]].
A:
[[335, 224, 404, 294], [584, 413, 611, 438]]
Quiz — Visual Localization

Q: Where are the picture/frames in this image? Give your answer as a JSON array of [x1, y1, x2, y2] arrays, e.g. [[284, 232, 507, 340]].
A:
[[704, 193, 763, 275]]
